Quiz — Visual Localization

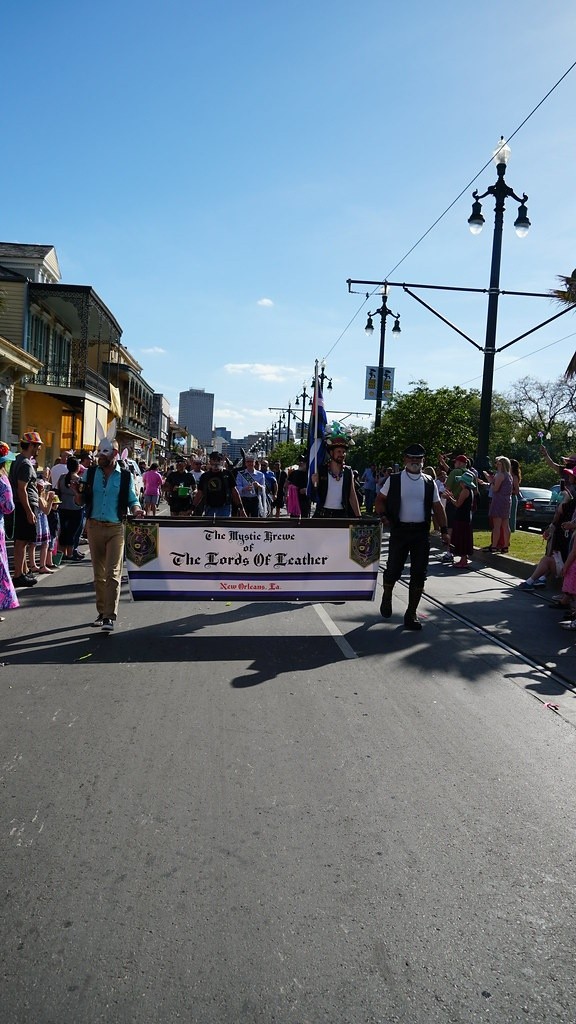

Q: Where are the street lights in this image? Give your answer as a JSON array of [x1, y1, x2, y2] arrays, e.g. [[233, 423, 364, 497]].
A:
[[567, 429, 574, 452], [526, 434, 533, 464], [248, 408, 286, 456], [294, 380, 313, 444], [468, 133, 532, 525], [511, 436, 515, 460], [310, 358, 334, 395], [364, 286, 404, 433], [281, 398, 298, 445], [546, 431, 551, 454]]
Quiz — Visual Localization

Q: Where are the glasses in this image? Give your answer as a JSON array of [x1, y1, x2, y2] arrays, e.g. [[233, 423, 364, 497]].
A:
[[260, 464, 267, 467], [210, 458, 221, 462], [493, 460, 499, 464], [275, 463, 281, 467]]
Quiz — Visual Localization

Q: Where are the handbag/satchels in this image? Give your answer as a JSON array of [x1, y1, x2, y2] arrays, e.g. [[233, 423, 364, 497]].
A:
[[517, 492, 523, 500]]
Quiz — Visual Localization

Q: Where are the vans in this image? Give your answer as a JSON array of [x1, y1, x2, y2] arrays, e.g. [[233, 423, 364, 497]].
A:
[[127, 460, 146, 507]]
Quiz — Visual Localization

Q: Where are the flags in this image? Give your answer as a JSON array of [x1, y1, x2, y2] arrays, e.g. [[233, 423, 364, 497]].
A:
[[306, 378, 327, 496]]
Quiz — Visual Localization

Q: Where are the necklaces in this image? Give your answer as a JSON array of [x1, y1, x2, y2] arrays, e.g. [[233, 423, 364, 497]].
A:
[[328, 459, 343, 481], [405, 470, 421, 481]]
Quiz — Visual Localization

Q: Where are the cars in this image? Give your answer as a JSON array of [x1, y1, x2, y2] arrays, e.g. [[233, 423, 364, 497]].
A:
[[516, 487, 557, 533]]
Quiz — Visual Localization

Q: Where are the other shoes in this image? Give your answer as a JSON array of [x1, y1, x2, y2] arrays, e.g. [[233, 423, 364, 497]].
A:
[[13, 536, 93, 587], [440, 541, 510, 567], [375, 581, 576, 634]]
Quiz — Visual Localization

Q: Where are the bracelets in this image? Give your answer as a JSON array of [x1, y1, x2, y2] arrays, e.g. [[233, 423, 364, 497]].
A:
[[543, 451, 548, 457], [549, 523, 555, 531], [440, 526, 449, 534]]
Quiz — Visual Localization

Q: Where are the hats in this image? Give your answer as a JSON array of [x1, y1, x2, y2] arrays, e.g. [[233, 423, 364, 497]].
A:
[[564, 466, 576, 476], [209, 452, 225, 462], [45, 495, 62, 504], [437, 471, 448, 479], [327, 437, 348, 454], [353, 470, 361, 477], [561, 454, 576, 464], [449, 455, 468, 463], [36, 475, 53, 486], [403, 443, 426, 460], [454, 472, 477, 488], [20, 432, 44, 444], [0, 441, 20, 464], [174, 455, 187, 463]]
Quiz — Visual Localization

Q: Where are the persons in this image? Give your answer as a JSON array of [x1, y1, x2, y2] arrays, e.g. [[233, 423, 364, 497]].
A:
[[164, 461, 198, 517], [179, 452, 248, 517], [376, 443, 449, 631], [514, 444, 576, 628], [0, 432, 146, 621], [236, 456, 265, 517], [309, 438, 361, 518], [67, 438, 145, 630], [363, 455, 521, 567], [141, 459, 310, 519]]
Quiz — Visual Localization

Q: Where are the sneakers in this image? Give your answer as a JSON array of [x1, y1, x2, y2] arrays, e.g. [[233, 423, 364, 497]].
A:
[[93, 612, 104, 625], [102, 618, 113, 630]]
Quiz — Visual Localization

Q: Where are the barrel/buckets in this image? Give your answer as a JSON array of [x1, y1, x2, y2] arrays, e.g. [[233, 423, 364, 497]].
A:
[[52, 550, 65, 566], [176, 487, 192, 497]]
